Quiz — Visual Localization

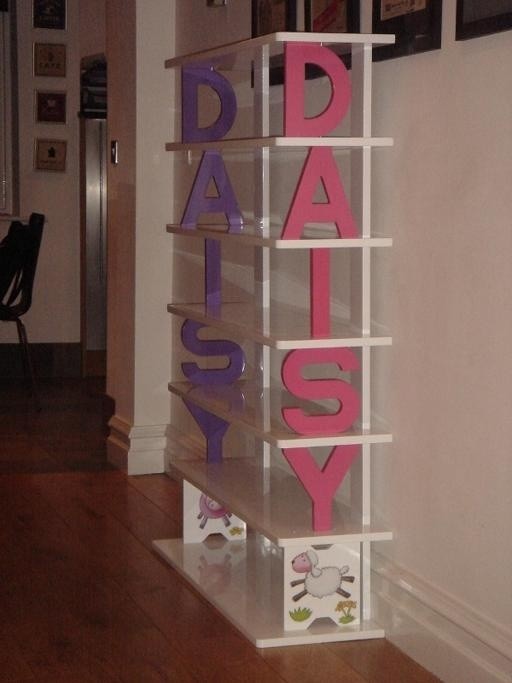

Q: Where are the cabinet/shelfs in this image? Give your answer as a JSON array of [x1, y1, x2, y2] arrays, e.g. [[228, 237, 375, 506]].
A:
[[149, 30, 399, 647]]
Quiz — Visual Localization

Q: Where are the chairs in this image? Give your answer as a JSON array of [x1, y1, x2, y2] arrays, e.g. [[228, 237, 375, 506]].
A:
[[1, 214, 46, 392]]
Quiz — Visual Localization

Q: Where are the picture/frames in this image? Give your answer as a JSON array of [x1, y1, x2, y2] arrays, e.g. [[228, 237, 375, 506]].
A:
[[31, 0, 69, 172], [250, 0, 512, 90]]
